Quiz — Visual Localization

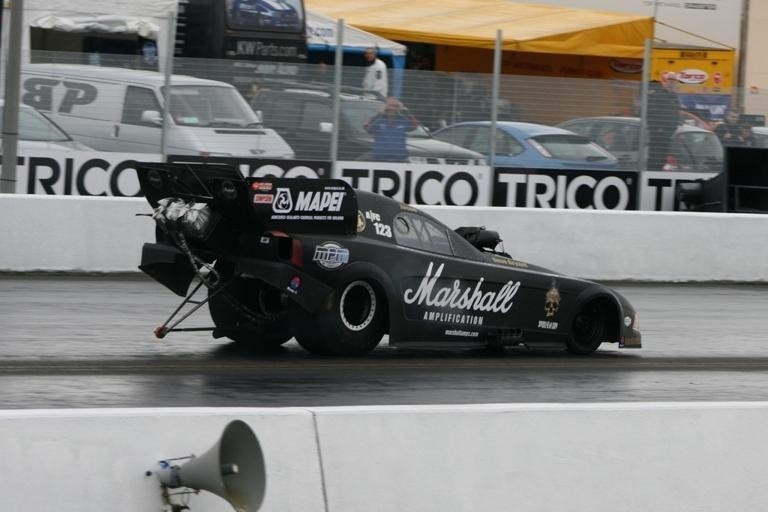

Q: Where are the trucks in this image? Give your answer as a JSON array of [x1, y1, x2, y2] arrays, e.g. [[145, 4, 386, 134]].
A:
[[23, 1, 311, 130]]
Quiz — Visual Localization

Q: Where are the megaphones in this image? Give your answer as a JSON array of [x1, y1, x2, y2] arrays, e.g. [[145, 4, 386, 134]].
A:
[[157, 420, 265, 511]]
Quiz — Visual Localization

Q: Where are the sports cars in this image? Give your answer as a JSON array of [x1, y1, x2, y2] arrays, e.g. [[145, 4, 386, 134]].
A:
[[130, 159, 643, 359]]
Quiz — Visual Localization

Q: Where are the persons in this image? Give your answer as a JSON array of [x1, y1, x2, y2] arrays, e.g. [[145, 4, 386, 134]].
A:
[[645, 79, 681, 172], [364, 96, 419, 164], [311, 61, 334, 84], [358, 47, 389, 101], [740, 123, 758, 148], [711, 107, 745, 147]]
